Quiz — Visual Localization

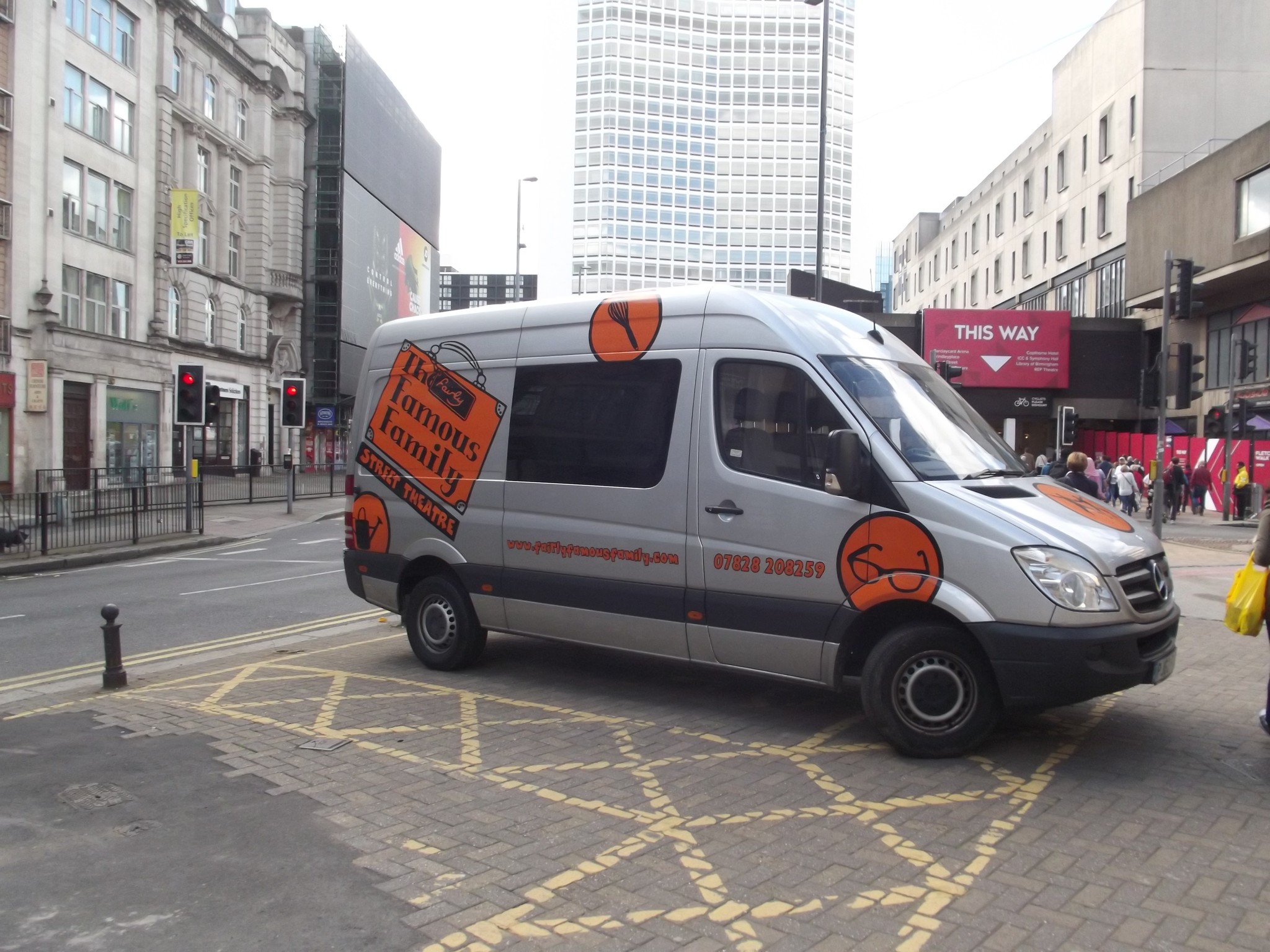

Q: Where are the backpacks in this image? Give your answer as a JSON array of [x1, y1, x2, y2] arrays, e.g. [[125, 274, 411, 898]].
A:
[[1048, 463, 1067, 480]]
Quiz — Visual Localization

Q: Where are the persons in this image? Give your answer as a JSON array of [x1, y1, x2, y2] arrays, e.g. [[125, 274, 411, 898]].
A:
[[1040, 454, 1056, 475], [1048, 448, 1071, 479], [1094, 453, 1174, 503], [1181, 464, 1196, 516], [1035, 450, 1048, 476], [1082, 456, 1107, 502], [1164, 456, 1184, 521], [1130, 464, 1142, 512], [1106, 461, 1119, 508], [1096, 455, 1112, 503], [1190, 460, 1213, 516], [1020, 447, 1035, 471], [1232, 461, 1249, 521], [1251, 482, 1270, 735], [1112, 457, 1130, 514], [1055, 451, 1099, 499], [1116, 464, 1139, 516]]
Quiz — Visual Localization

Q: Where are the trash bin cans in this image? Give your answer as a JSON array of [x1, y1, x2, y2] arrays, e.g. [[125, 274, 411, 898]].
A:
[[1250, 483, 1263, 518], [251, 448, 262, 478]]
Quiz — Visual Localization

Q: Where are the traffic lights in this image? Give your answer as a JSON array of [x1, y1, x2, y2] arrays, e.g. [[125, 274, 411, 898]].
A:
[[202, 384, 220, 427], [1239, 340, 1258, 379], [175, 363, 205, 425], [1238, 398, 1256, 438], [1062, 406, 1079, 445], [1176, 342, 1205, 409], [1176, 259, 1206, 319], [280, 377, 306, 428], [940, 362, 962, 392], [1207, 406, 1225, 437]]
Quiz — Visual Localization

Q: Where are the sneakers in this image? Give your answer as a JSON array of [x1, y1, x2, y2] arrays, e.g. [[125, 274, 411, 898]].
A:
[[1258, 708, 1270, 735]]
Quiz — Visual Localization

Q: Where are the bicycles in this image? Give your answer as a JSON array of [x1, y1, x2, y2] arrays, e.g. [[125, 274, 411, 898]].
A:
[[1145, 486, 1168, 523]]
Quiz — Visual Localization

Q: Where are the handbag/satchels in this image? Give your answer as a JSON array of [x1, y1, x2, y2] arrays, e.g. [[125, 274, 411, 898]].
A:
[[1134, 492, 1142, 513], [1223, 550, 1269, 637]]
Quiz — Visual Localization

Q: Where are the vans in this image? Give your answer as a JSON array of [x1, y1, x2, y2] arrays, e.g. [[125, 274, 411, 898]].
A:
[[343, 281, 1183, 759]]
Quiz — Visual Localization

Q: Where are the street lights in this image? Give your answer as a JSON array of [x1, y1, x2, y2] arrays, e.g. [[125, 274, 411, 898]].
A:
[[578, 265, 592, 296], [804, 0, 829, 302], [515, 176, 537, 303]]
